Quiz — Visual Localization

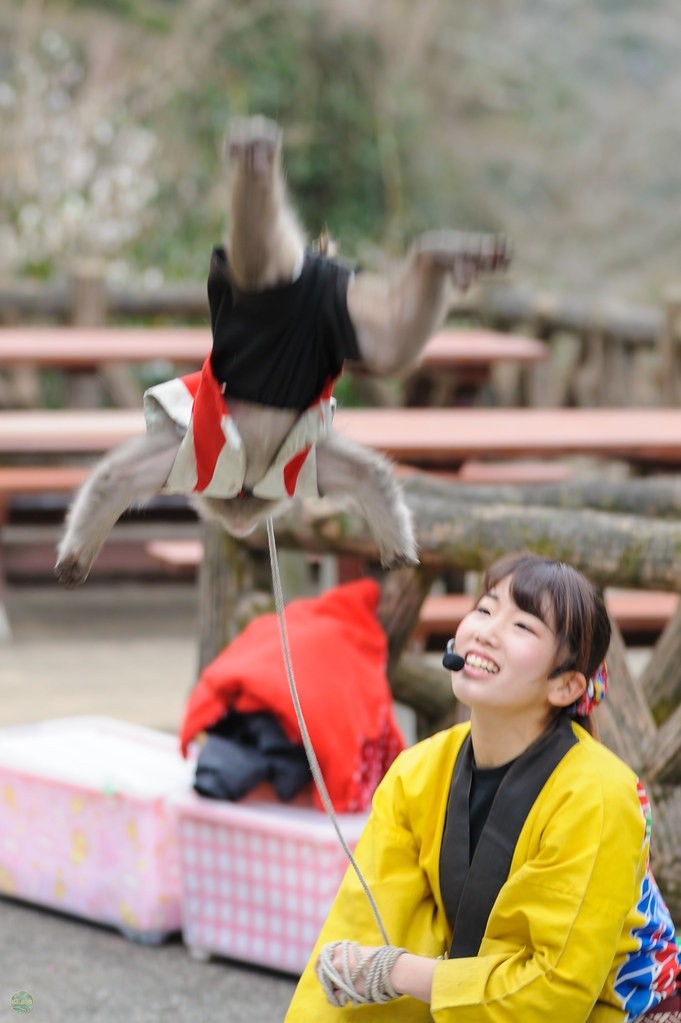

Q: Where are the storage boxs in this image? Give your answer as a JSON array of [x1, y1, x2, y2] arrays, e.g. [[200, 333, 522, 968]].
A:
[[0, 715, 201, 948], [177, 791, 369, 978]]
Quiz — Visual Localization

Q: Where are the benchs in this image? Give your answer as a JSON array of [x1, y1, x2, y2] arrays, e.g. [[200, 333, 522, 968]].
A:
[[0, 459, 569, 521], [407, 580, 681, 640]]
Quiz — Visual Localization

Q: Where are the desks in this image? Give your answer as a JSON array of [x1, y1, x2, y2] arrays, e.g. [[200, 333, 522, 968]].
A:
[[0, 322, 552, 401], [4, 400, 680, 457]]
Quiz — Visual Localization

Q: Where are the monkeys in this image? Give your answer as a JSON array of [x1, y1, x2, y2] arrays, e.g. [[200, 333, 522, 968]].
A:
[[54, 111, 508, 589]]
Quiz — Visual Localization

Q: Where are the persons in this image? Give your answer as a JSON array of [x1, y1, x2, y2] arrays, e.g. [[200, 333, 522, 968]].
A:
[[285, 553, 681, 1023]]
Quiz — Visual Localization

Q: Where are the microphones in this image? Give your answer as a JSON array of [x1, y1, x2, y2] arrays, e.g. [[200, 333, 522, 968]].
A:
[[442, 638, 465, 671]]
